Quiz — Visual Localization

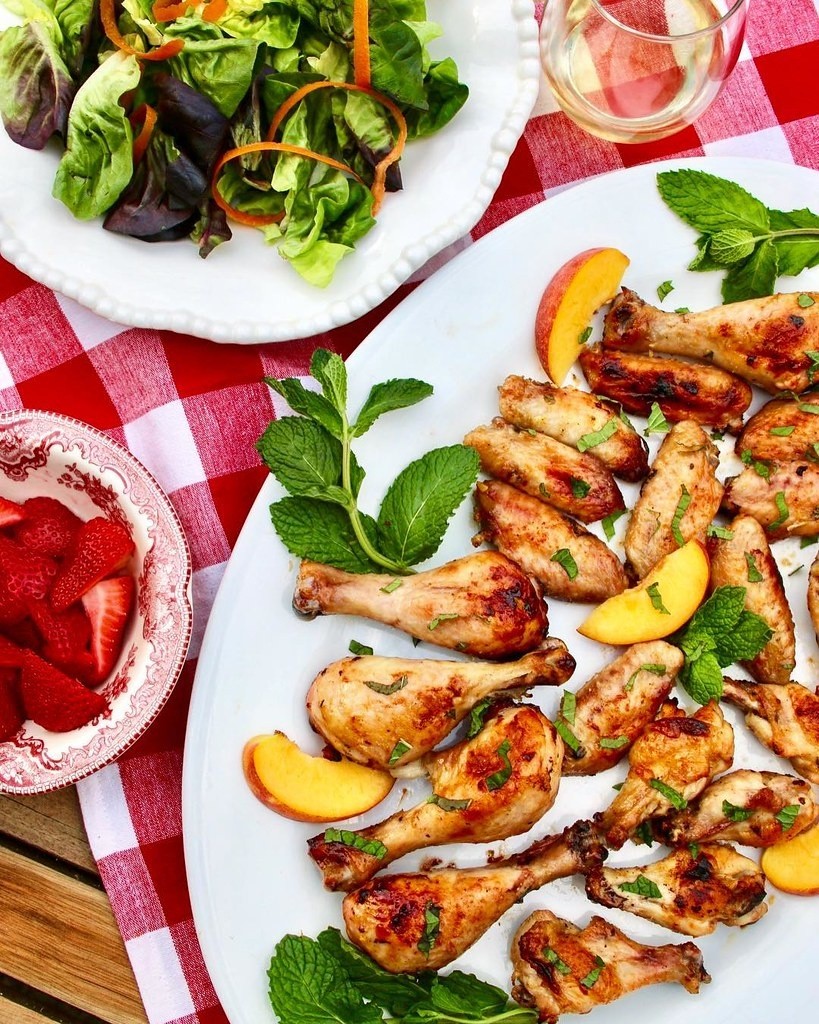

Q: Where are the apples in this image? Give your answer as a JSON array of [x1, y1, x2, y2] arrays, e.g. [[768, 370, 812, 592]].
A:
[[536, 247, 631, 387], [760, 822, 818, 895], [576, 537, 710, 646], [242, 730, 397, 823]]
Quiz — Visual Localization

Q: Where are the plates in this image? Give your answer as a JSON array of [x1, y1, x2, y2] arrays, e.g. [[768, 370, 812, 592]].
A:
[[1, 409, 198, 798], [1, 0, 539, 345], [180, 156, 818, 1023]]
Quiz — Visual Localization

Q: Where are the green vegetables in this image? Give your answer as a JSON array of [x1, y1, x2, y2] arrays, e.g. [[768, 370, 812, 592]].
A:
[[0, 0, 469, 291], [254, 169, 819, 1024]]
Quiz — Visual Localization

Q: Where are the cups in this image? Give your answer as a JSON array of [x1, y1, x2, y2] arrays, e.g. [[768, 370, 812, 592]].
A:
[[540, 0, 750, 143]]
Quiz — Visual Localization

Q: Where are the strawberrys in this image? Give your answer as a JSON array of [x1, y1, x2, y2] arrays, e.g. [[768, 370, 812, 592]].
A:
[[0, 495, 133, 744]]
